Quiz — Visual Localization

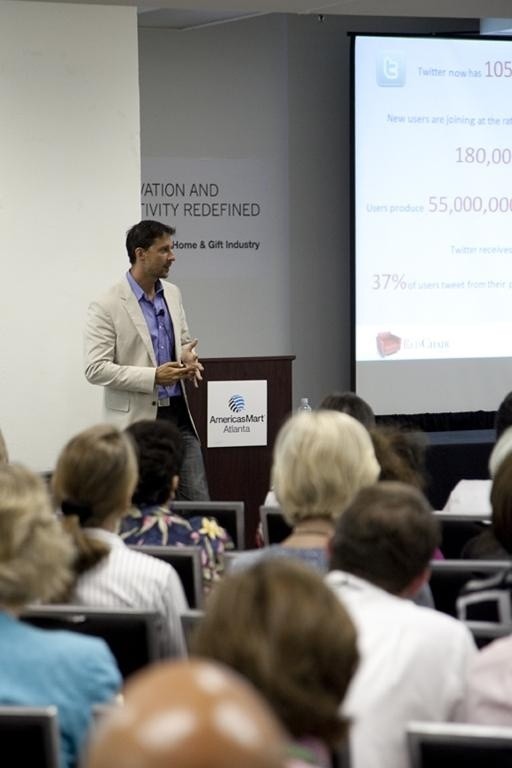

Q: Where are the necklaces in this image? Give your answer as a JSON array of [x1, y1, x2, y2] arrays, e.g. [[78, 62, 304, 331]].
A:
[[292, 526, 333, 537]]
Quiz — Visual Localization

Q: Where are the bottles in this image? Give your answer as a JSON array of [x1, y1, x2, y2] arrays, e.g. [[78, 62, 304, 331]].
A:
[[297, 398, 310, 414]]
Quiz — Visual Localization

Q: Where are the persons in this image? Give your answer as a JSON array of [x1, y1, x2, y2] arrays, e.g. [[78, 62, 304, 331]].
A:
[[2, 465, 126, 768], [442, 390, 512, 516], [459, 424, 512, 561], [210, 408, 435, 603], [116, 419, 235, 600], [188, 548, 358, 768], [83, 657, 296, 768], [318, 480, 481, 768], [479, 447, 511, 727], [256, 392, 377, 547], [34, 424, 190, 665], [367, 429, 443, 560], [85, 219, 210, 501]]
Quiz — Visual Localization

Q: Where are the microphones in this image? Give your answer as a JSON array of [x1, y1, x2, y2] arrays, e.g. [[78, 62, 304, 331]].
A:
[[156, 309, 168, 324]]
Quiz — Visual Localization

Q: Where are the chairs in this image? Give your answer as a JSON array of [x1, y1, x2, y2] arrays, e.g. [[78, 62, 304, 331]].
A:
[[126, 543, 204, 609], [166, 499, 243, 552], [430, 510, 495, 561], [17, 604, 163, 680], [404, 721, 512, 767], [0, 704, 64, 767], [180, 610, 209, 657], [427, 560, 512, 651], [258, 505, 296, 547]]
